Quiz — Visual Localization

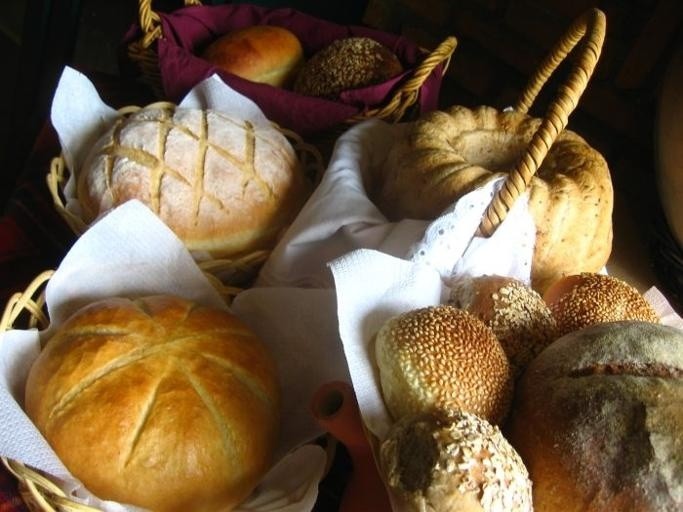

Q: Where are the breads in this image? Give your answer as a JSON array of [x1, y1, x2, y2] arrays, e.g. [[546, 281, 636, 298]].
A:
[[75, 104, 301, 266], [200, 24, 306, 89], [25, 294, 283, 512], [496, 318, 683, 512], [377, 107, 615, 295], [295, 34, 403, 100], [365, 304, 513, 432], [542, 271, 662, 335], [453, 273, 562, 378], [372, 408, 533, 512]]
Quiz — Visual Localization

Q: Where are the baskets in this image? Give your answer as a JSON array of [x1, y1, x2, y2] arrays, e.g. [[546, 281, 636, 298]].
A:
[[125, 0, 457, 159], [1, 0, 607, 512], [0, 269, 337, 512], [46, 101, 326, 287]]
